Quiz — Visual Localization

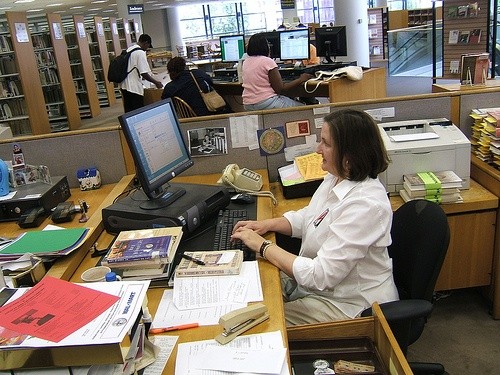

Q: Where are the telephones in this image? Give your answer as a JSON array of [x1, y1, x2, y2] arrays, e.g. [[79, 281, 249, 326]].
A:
[[216, 163, 263, 191]]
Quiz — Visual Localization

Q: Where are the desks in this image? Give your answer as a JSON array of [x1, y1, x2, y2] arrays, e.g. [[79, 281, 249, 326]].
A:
[[142, 67, 387, 106]]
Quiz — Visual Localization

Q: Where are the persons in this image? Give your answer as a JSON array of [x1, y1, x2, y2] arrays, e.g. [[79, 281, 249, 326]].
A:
[[120, 34, 163, 113], [284, 43, 321, 105], [241, 34, 311, 111], [160, 57, 231, 119], [230, 109, 399, 326]]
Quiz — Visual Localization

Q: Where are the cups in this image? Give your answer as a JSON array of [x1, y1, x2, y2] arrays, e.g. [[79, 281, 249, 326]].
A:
[[81, 266, 121, 283]]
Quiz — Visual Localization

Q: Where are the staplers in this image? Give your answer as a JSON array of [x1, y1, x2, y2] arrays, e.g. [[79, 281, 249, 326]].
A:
[[215, 302, 270, 345]]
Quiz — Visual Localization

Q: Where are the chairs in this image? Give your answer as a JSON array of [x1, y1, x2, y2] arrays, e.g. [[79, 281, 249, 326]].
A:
[[361, 199, 451, 375], [172, 96, 197, 119]]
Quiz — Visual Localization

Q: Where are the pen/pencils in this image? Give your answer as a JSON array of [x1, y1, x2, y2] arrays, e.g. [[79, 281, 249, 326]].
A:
[[179, 252, 205, 266], [149, 323, 198, 334]]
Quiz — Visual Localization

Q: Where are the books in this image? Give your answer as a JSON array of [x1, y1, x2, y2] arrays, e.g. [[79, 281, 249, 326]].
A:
[[101, 226, 183, 281], [176, 249, 243, 277], [400, 170, 462, 203]]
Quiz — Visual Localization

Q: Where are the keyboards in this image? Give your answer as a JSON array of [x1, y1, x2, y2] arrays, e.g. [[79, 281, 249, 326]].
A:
[[211, 76, 235, 82], [213, 209, 256, 261]]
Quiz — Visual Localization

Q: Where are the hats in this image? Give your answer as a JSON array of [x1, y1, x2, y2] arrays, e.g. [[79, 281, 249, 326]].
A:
[[140, 34, 154, 48]]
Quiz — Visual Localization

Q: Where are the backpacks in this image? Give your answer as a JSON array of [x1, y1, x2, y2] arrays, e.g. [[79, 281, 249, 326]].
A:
[[107, 45, 146, 83]]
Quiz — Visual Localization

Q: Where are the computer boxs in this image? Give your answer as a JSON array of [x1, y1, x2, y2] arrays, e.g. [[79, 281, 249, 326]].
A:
[[214, 68, 239, 78], [0, 175, 71, 222], [278, 61, 358, 79], [101, 182, 230, 241]]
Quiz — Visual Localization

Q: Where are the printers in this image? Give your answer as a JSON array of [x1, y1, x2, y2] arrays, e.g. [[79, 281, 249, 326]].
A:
[[375, 117, 471, 197]]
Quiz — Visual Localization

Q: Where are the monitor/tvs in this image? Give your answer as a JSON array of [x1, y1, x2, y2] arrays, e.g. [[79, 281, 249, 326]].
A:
[[315, 26, 347, 64], [261, 28, 310, 68], [116, 97, 194, 210], [220, 34, 246, 70]]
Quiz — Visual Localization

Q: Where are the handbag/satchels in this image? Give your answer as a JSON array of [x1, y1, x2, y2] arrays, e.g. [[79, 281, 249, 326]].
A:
[[304, 66, 363, 93], [189, 71, 227, 112]]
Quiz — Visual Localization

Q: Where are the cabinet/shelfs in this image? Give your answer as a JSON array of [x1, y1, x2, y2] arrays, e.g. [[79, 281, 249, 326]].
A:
[[382, 7, 442, 43], [0, 10, 137, 135], [390, 153, 500, 319], [0, 171, 416, 375]]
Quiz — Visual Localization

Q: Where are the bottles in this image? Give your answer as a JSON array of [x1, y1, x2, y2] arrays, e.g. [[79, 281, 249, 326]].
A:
[[142, 308, 152, 323], [106, 272, 116, 282]]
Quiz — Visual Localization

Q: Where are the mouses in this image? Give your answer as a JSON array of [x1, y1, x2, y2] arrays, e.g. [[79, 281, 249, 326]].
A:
[[230, 194, 256, 205], [232, 78, 238, 82]]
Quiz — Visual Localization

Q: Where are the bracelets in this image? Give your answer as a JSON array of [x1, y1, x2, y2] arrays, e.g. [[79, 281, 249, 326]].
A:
[[260, 240, 275, 260]]
